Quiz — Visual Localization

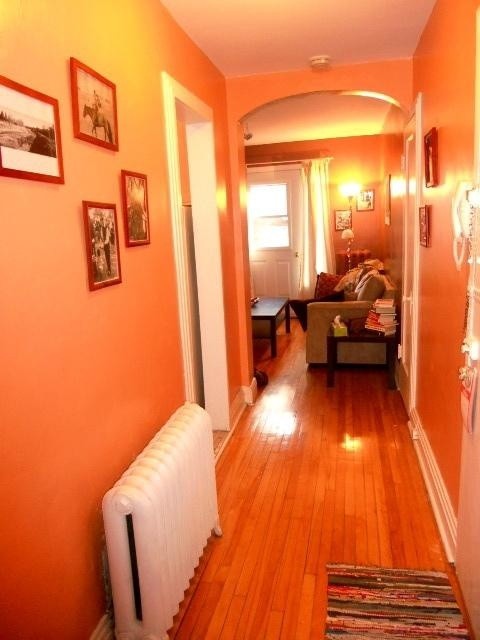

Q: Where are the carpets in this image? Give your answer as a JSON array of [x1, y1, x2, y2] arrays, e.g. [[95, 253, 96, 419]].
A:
[[325, 563, 471, 640]]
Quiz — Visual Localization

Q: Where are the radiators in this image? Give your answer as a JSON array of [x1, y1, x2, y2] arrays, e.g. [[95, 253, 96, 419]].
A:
[[102, 403, 223, 640]]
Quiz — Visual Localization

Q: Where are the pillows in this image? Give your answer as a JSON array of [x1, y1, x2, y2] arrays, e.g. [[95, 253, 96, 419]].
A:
[[288, 268, 363, 333]]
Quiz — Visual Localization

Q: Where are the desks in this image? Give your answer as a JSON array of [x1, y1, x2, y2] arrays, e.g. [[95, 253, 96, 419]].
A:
[[326, 317, 396, 389], [250, 296, 290, 357]]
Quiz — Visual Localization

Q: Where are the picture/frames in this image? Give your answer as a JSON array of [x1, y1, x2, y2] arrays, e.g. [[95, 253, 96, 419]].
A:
[[81, 200, 122, 292], [335, 210, 352, 231], [424, 127, 439, 189], [69, 57, 119, 152], [119, 170, 151, 248], [0, 75, 64, 185], [356, 190, 374, 212], [419, 205, 430, 249], [383, 174, 392, 227]]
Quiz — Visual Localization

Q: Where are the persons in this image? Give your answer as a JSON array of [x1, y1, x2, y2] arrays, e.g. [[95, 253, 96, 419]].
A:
[[92, 90, 102, 113], [99, 218, 112, 276]]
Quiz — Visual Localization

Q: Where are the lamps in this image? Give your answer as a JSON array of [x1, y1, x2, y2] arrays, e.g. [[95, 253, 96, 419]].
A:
[[336, 182, 362, 210], [342, 228, 354, 274], [242, 122, 253, 140]]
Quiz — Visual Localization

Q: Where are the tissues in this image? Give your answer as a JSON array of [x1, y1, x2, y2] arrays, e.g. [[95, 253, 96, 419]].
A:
[[330, 313, 349, 336]]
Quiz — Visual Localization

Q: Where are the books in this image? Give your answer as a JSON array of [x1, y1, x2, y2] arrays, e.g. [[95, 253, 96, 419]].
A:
[[364, 297, 399, 333]]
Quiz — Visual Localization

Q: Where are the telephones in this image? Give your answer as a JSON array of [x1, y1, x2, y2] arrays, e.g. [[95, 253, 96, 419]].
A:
[[452, 181, 473, 273]]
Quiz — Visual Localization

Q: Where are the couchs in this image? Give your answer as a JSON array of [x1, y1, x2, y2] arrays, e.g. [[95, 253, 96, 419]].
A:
[[306, 259, 395, 365]]
